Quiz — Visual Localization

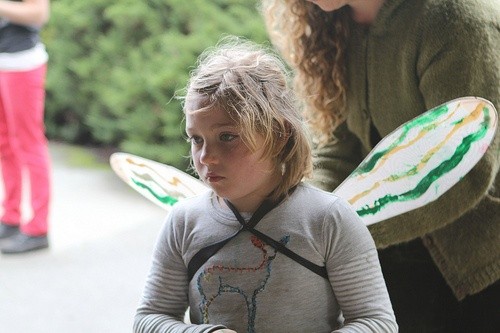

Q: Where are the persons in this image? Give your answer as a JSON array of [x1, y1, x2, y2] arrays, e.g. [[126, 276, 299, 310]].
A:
[[131, 32, 400, 333], [258, 1, 500, 333], [0, 0, 53, 253]]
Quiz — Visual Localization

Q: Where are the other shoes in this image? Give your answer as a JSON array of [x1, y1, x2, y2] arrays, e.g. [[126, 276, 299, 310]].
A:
[[0, 232, 49, 254], [0, 222, 19, 238]]
